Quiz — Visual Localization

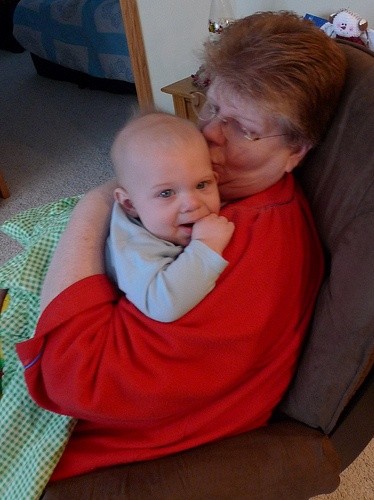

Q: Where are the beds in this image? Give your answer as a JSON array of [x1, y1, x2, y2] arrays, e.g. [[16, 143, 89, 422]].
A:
[[13, 0, 138, 96]]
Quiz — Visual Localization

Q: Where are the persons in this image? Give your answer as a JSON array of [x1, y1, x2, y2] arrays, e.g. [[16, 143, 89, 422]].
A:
[[16, 10, 347, 481], [104, 113, 235, 322]]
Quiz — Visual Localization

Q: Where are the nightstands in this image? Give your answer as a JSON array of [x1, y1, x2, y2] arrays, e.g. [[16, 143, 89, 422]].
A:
[[161, 77, 208, 124]]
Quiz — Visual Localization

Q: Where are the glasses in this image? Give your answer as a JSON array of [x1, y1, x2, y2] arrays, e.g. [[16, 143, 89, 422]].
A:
[[188, 83, 292, 146]]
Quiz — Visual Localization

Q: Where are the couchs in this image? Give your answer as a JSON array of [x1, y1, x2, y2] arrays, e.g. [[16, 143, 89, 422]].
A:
[[40, 39, 374, 500]]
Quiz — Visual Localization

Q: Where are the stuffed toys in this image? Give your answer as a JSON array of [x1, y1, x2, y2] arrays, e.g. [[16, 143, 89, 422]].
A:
[[329, 8, 368, 46]]
